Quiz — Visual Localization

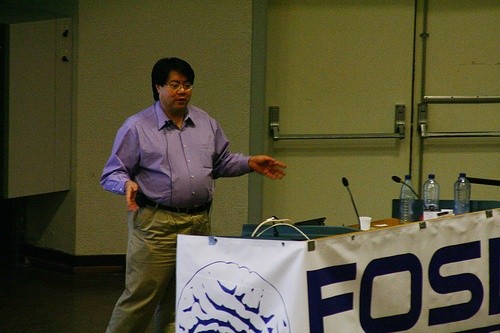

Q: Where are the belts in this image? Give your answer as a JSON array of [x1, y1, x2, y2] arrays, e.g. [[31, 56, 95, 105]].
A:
[[140, 195, 211, 214]]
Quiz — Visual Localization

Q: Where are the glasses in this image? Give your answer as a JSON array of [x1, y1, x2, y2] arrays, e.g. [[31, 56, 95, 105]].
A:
[[164, 82, 193, 91]]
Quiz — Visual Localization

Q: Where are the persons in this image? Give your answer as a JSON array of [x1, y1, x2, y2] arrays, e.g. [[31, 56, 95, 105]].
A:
[[99, 56, 289, 333]]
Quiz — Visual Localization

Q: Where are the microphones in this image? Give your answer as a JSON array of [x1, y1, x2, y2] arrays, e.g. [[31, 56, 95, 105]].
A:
[[342, 177, 361, 230], [392, 175, 428, 210]]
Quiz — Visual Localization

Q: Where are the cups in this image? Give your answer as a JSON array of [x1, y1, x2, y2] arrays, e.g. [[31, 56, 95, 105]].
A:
[[359, 217, 372, 232]]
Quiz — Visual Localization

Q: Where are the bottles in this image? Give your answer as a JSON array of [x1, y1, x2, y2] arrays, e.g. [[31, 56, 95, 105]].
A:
[[423, 173, 440, 212], [452, 172, 471, 215], [400, 176, 416, 221]]
